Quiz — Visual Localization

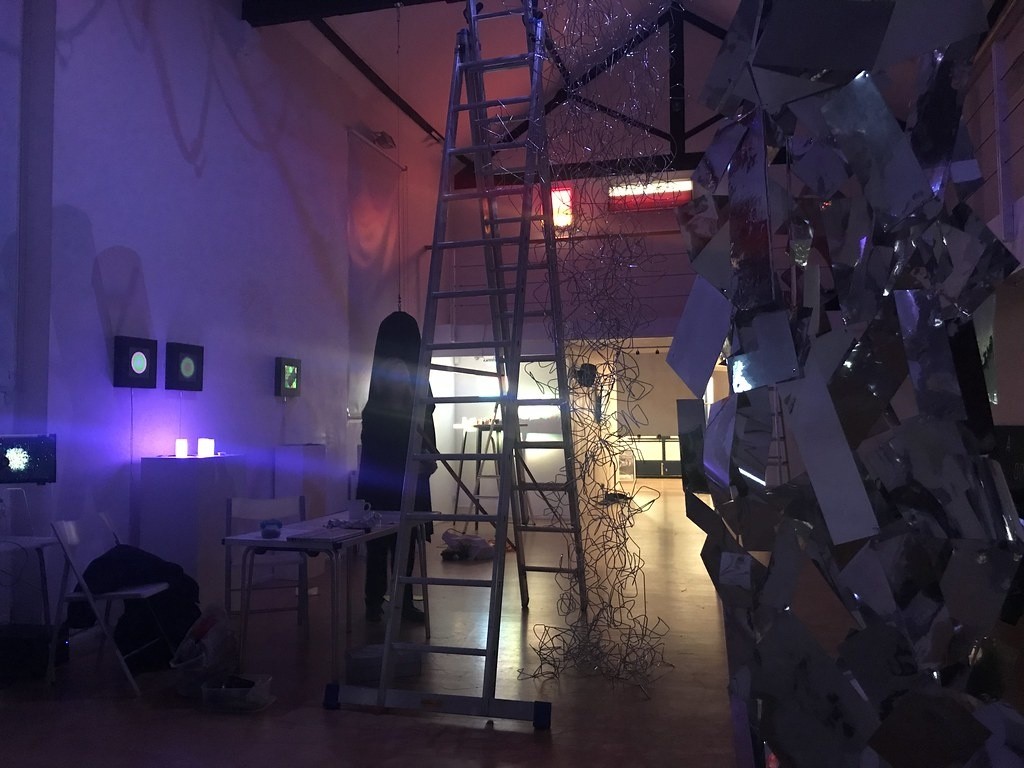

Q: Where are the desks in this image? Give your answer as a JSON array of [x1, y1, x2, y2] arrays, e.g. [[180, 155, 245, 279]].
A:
[[452, 423, 535, 530], [222, 508, 443, 688], [0, 536, 60, 669]]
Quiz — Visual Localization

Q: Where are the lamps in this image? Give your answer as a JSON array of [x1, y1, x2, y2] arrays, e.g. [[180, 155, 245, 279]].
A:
[[175, 439, 188, 458], [197, 438, 215, 458]]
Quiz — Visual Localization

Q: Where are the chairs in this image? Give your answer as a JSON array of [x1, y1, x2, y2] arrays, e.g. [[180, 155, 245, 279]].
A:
[[224, 494, 306, 632], [50, 511, 176, 705]]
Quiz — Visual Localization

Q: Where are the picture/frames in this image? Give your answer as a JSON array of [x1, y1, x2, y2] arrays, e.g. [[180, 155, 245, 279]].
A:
[[114, 335, 157, 389], [275, 357, 302, 397], [165, 342, 204, 391]]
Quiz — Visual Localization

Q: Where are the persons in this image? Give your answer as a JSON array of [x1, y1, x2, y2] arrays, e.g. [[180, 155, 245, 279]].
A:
[[362, 359, 436, 622]]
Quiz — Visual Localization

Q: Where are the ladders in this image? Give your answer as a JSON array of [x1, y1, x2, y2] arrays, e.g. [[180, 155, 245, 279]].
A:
[[320, 0, 593, 732]]
